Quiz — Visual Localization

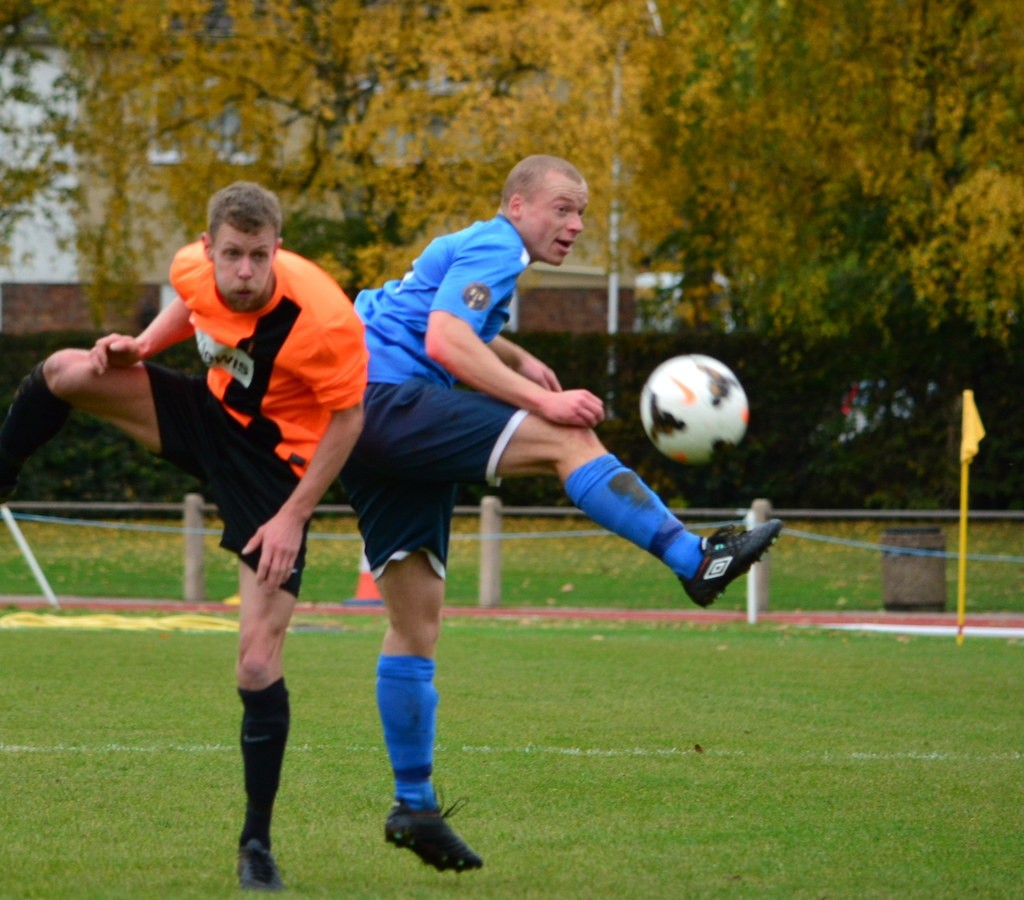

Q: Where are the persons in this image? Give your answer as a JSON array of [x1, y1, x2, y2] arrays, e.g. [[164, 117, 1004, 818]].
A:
[[1, 178, 365, 892], [333, 155, 786, 874]]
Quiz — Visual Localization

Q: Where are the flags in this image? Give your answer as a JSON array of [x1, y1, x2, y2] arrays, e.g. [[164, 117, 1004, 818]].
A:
[[960, 390, 985, 463]]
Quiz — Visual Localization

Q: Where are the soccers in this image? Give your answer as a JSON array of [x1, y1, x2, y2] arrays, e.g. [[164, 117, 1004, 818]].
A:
[[638, 353, 750, 467]]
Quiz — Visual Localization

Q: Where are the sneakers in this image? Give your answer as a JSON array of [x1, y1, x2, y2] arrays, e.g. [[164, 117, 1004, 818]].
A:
[[234, 837, 285, 892], [677, 518, 783, 608], [382, 795, 484, 873]]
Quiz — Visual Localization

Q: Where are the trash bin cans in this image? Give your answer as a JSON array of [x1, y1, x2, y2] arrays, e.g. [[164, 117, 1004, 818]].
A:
[[879, 527, 947, 613]]
